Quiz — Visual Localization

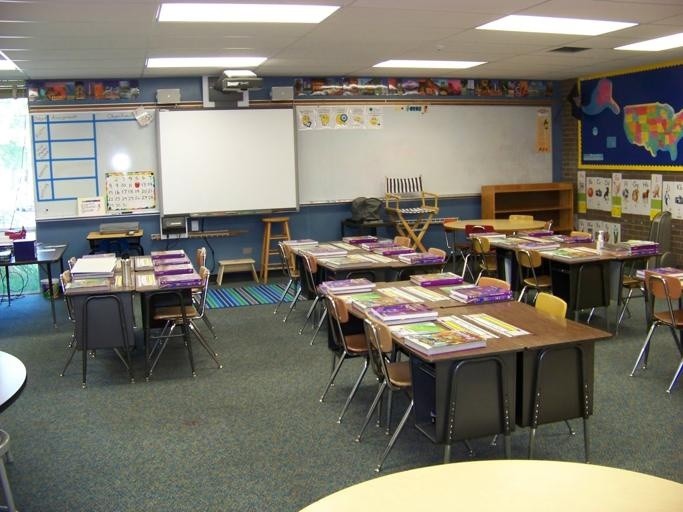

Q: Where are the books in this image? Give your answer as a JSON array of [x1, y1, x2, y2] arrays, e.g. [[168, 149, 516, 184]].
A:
[[465, 231, 660, 261], [319, 272, 532, 358], [636, 266, 682, 282], [65, 249, 205, 295], [281, 231, 442, 265]]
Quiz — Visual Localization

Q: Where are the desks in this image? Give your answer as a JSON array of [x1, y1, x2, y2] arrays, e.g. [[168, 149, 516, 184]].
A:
[[0, 243, 68, 329], [0, 351, 28, 512], [298, 459, 682, 511], [60, 228, 241, 388]]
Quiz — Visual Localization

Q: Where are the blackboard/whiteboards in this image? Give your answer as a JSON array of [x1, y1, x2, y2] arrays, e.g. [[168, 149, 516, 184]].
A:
[[155, 106, 300, 217], [29, 100, 555, 222]]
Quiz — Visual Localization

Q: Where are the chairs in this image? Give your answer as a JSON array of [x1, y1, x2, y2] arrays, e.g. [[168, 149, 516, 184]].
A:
[[570, 230, 592, 240], [385, 175, 440, 253]]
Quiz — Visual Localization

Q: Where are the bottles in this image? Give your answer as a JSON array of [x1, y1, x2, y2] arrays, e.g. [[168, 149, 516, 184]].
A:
[[121, 253, 132, 286], [596, 229, 604, 251]]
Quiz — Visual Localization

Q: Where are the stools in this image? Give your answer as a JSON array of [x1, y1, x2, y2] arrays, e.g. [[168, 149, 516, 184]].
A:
[[259, 217, 296, 284], [216, 258, 260, 286]]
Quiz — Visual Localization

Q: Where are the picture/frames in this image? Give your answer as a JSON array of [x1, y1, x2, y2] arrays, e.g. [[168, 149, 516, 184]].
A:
[[77, 197, 105, 217]]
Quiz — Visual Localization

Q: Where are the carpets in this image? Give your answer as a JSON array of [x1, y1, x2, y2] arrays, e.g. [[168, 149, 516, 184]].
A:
[[195, 281, 308, 310]]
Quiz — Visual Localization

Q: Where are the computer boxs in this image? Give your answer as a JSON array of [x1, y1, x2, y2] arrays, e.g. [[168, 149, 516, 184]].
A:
[[13, 239, 37, 261]]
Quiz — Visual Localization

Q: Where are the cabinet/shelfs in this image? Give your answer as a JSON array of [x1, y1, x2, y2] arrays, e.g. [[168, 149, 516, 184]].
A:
[[481, 183, 574, 235]]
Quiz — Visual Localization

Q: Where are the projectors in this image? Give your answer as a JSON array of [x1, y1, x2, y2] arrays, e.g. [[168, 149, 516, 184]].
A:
[[215, 78, 263, 93]]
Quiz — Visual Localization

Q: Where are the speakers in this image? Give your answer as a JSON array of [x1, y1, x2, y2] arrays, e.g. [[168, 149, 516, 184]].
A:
[[271, 86, 294, 101], [156, 88, 181, 104]]
